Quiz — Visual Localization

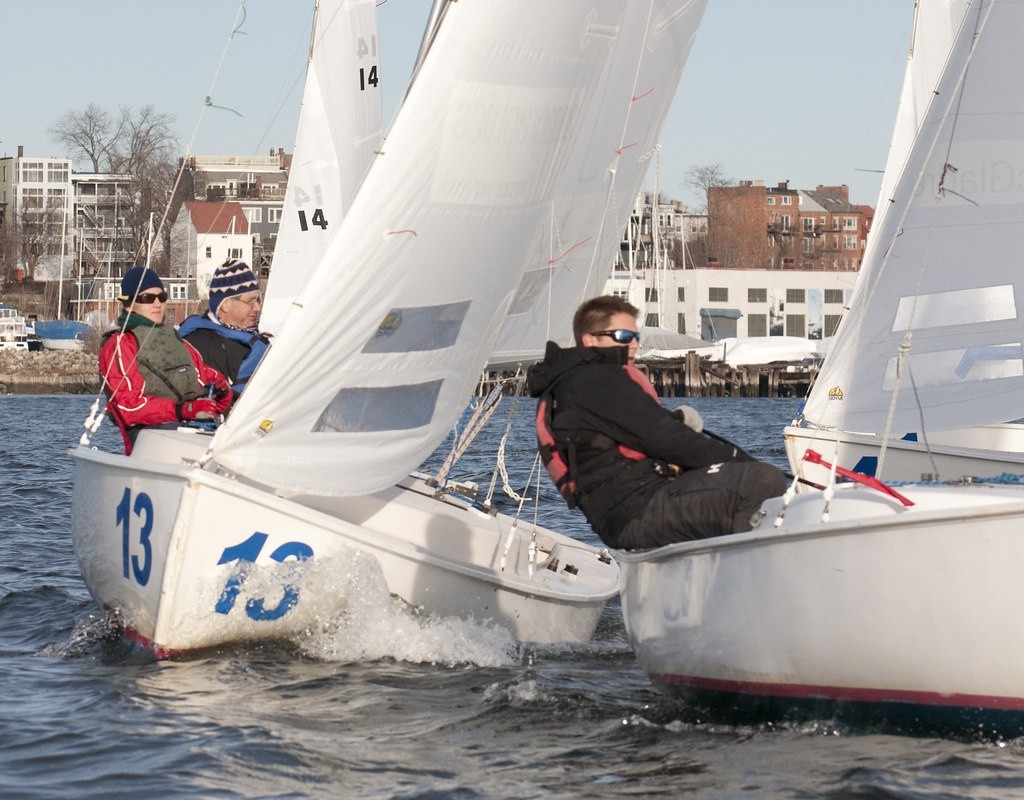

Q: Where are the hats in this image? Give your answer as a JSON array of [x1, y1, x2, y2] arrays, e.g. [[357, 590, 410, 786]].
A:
[[209, 259, 260, 320], [122, 266, 164, 307]]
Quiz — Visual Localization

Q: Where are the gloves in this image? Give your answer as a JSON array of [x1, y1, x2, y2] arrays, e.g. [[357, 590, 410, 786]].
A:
[[673, 404, 703, 433], [180, 393, 224, 422], [203, 378, 229, 391]]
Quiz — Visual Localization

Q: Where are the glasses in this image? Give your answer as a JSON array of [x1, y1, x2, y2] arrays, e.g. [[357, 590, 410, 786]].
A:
[[590, 333, 639, 344], [232, 297, 262, 309], [125, 291, 167, 304]]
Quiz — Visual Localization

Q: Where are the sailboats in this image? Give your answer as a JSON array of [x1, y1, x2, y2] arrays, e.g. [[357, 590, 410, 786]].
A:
[[623, 140, 724, 364], [607, 1, 1023, 735], [64, 0, 706, 662], [33, 183, 112, 352]]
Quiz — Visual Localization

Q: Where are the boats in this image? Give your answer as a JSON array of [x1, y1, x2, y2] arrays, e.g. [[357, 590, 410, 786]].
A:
[[1, 304, 29, 353]]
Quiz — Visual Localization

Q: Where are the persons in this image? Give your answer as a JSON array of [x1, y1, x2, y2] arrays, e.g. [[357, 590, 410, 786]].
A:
[[524, 294, 788, 551], [96, 262, 234, 457], [175, 258, 275, 433]]
